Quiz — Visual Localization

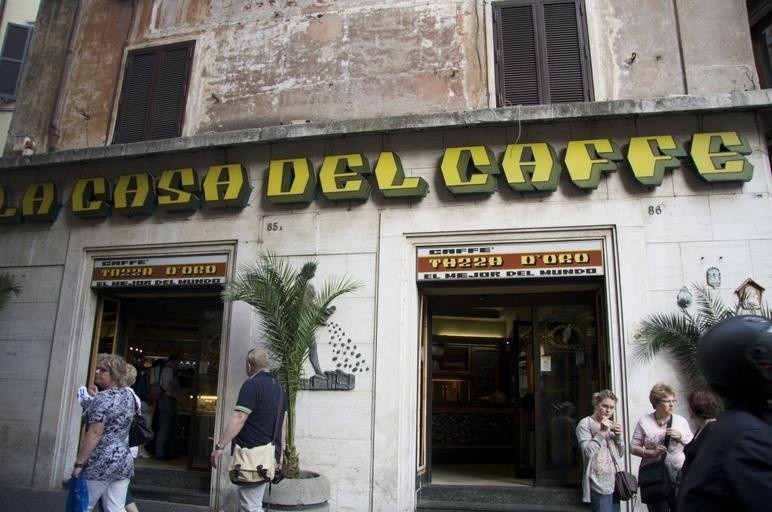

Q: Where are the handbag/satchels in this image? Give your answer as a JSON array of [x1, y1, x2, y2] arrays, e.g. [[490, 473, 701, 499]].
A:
[[129, 414, 155, 448], [616, 471, 638, 501], [638, 460, 672, 504], [227, 441, 276, 486], [147, 382, 164, 402]]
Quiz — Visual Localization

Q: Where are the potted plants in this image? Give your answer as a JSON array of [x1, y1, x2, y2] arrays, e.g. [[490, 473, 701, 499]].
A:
[[219, 250, 368, 512]]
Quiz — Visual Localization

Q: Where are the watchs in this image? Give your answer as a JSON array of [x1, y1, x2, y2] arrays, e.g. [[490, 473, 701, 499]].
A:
[[215, 444, 224, 450]]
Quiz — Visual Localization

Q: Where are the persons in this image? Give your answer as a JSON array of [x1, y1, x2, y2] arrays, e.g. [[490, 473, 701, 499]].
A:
[[88, 361, 141, 511], [135, 358, 166, 457], [575, 389, 624, 511], [671, 389, 720, 472], [155, 352, 182, 462], [73, 352, 137, 512], [673, 314, 771, 511], [630, 384, 694, 511], [209, 347, 290, 512]]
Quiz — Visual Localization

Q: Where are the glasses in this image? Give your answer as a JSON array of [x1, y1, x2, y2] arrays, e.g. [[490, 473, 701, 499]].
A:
[[658, 400, 677, 405]]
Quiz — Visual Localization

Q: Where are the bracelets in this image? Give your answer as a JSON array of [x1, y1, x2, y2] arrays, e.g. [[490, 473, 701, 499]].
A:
[[75, 463, 84, 469]]
